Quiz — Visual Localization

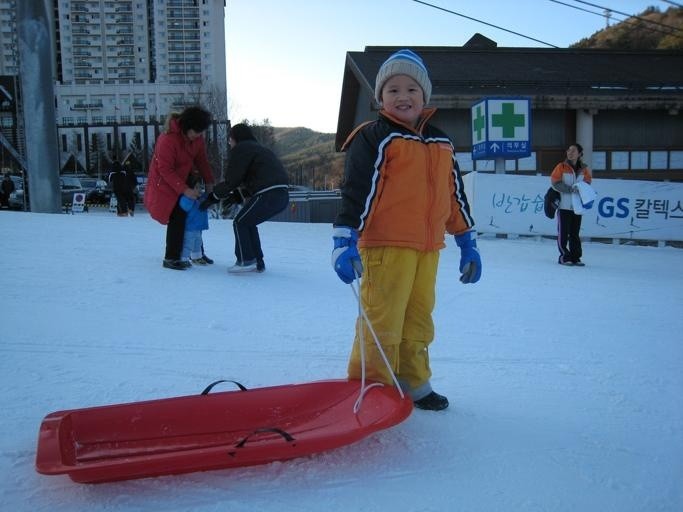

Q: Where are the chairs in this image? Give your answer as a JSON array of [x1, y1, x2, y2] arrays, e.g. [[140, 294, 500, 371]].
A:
[[162, 255, 213, 270], [563, 261, 585, 267], [413, 390, 450, 411], [226, 260, 265, 275]]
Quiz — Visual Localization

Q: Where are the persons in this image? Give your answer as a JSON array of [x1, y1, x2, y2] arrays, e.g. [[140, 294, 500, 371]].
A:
[[330, 44, 484, 412], [144, 106, 217, 272], [122, 160, 140, 216], [108, 160, 128, 216], [1, 173, 15, 207], [198, 122, 290, 274], [549, 142, 592, 267], [179, 173, 214, 266]]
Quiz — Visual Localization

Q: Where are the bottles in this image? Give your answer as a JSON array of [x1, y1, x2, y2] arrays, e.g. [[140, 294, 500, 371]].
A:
[[185, 172, 198, 190], [372, 48, 432, 107]]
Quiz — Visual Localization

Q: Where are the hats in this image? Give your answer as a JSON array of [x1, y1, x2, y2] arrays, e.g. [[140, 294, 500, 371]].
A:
[[0, 174, 148, 212]]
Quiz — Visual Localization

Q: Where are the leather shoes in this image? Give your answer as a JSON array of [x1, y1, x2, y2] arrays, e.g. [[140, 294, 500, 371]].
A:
[[453, 228, 482, 285], [221, 187, 245, 210], [328, 225, 360, 284], [197, 192, 220, 213]]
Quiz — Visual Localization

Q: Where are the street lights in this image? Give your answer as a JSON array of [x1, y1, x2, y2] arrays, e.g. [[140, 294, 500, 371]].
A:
[[543, 185, 562, 220]]
